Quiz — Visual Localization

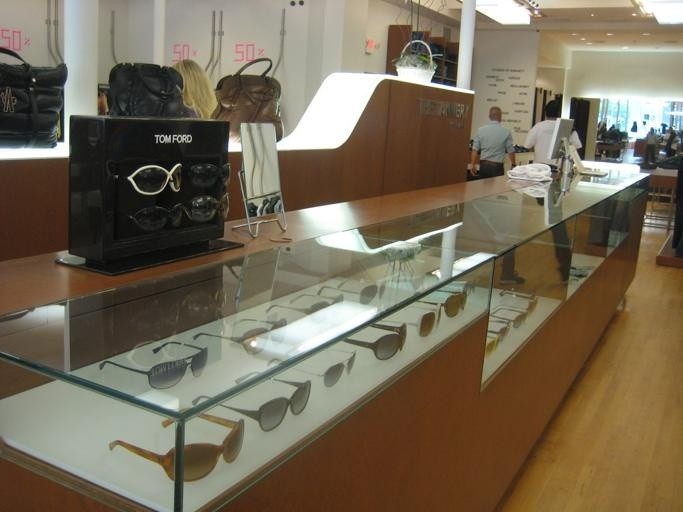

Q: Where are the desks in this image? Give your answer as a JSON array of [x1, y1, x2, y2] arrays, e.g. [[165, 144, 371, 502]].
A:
[[638, 168, 679, 231]]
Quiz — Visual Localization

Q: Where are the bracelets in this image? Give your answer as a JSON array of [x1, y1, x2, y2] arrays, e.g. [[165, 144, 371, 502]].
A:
[[510, 164, 515, 167]]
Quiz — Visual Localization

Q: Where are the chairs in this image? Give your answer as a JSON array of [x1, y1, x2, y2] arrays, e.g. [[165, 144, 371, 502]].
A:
[[2, 52, 286, 148]]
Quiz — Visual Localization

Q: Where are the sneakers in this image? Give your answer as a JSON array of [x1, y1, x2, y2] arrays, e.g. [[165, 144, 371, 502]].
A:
[[498, 268, 588, 285]]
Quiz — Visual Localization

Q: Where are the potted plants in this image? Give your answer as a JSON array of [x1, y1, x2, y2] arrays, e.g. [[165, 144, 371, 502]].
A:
[[391, 41, 437, 84]]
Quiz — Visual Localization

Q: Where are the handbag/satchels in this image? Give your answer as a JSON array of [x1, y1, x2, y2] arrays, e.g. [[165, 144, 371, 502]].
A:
[[0, 47, 70, 149], [210, 56, 284, 143], [106, 62, 185, 117]]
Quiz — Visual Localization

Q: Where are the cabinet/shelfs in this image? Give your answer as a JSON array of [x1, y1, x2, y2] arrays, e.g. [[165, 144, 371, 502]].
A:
[[386, 23, 460, 88]]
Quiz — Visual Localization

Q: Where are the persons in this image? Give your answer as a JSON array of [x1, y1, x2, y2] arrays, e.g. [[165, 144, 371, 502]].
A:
[[470, 106, 516, 180], [643, 126, 683, 168], [536, 178, 584, 283], [479, 195, 526, 284], [525, 100, 583, 163], [171, 59, 219, 117], [595, 122, 621, 158]]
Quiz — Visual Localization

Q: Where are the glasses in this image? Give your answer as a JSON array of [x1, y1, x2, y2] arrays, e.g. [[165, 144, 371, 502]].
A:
[[266, 280, 467, 360], [126, 201, 184, 231], [183, 190, 231, 224], [99, 319, 357, 482], [485, 290, 540, 352], [124, 160, 185, 197], [188, 163, 232, 190]]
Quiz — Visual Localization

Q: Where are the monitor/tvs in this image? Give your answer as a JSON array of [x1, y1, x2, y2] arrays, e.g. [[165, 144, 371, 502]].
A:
[[544, 174, 572, 226], [545, 117, 583, 173]]
